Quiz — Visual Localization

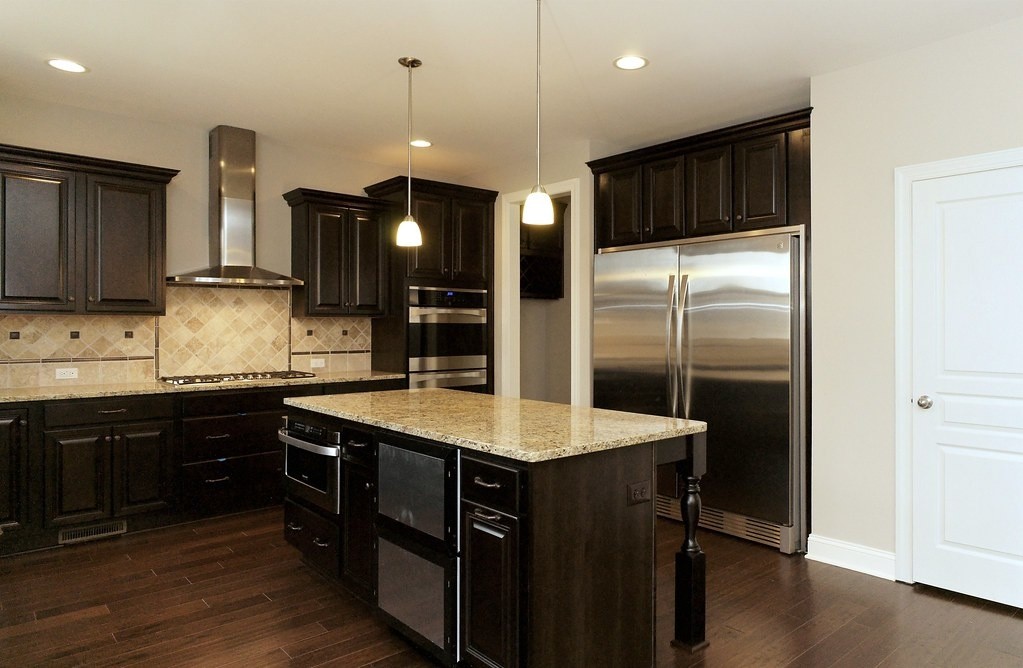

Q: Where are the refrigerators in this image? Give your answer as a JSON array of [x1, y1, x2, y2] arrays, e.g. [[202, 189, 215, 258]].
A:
[[593, 222, 809, 555]]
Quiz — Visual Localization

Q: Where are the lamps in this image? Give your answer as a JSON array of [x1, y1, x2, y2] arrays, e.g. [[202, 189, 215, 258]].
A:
[[521, 0, 554, 226], [396, 56, 425, 248]]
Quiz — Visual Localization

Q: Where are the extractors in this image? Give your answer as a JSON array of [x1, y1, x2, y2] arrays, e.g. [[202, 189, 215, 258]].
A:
[[165, 266, 305, 290]]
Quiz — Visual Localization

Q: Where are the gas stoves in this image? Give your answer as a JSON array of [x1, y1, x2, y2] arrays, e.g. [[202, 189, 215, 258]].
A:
[[161, 371, 316, 386]]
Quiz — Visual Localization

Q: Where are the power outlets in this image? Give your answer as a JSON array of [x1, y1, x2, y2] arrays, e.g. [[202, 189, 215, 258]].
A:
[[55, 368, 79, 379], [310, 358, 326, 367]]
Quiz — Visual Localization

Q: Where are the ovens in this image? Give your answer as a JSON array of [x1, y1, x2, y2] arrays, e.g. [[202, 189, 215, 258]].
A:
[[277, 416, 343, 519], [406, 285, 489, 394]]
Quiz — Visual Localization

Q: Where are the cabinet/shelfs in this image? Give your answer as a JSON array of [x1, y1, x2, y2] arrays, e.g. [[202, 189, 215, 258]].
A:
[[281, 188, 400, 318], [686, 131, 787, 238], [0, 401, 35, 559], [37, 392, 175, 552], [520, 202, 566, 299], [595, 153, 686, 247], [177, 388, 278, 524], [0, 143, 180, 316], [408, 179, 488, 289], [459, 447, 526, 667]]
